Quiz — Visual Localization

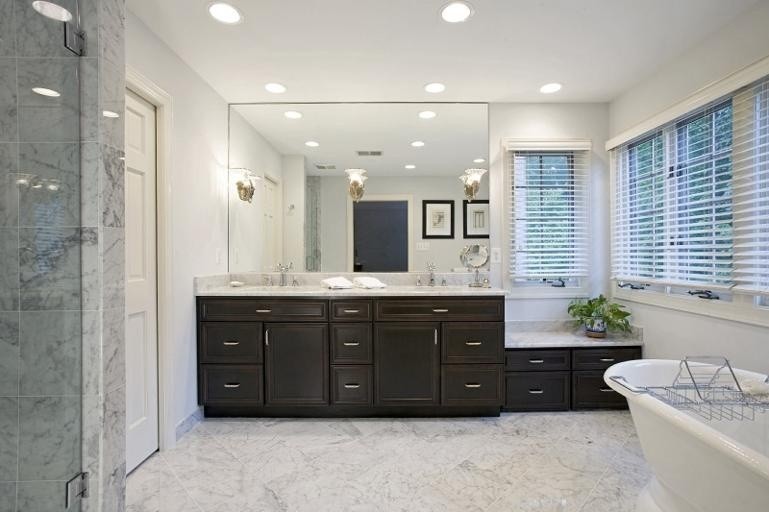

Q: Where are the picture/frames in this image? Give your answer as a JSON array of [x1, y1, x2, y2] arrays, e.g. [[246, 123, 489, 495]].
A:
[[462, 199, 489, 239], [421, 199, 456, 240]]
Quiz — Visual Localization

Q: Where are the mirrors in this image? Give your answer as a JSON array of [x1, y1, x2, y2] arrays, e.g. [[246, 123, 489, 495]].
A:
[[460, 241, 489, 288], [227, 101, 491, 275]]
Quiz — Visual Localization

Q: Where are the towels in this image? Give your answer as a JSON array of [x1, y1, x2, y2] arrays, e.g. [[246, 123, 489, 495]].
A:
[[322, 276, 353, 290], [351, 276, 388, 291]]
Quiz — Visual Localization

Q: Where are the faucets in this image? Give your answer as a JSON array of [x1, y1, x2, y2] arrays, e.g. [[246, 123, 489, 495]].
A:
[[276, 261, 292, 286], [425, 262, 440, 286]]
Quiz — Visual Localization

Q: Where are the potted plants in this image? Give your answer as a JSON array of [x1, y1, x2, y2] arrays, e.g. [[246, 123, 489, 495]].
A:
[[566, 293, 633, 339]]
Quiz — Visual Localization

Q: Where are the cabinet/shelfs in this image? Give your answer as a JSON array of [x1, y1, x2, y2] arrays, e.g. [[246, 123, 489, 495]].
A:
[[373, 295, 507, 418], [569, 342, 644, 411], [502, 346, 574, 413], [194, 298, 329, 420], [329, 298, 374, 418]]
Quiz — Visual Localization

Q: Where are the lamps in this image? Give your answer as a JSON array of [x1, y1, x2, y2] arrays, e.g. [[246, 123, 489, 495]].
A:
[[458, 167, 487, 203], [230, 167, 262, 204], [345, 167, 368, 203]]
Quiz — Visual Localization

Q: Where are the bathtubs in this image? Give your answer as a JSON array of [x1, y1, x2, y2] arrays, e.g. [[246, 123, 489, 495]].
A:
[[604, 358, 769, 512]]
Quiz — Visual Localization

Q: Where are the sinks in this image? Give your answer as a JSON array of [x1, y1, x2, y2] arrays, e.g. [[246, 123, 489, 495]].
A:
[[402, 287, 462, 291], [244, 286, 321, 292]]
